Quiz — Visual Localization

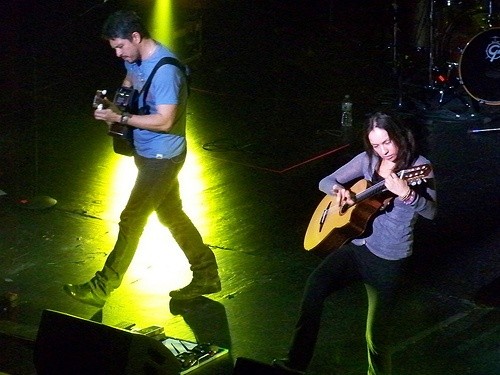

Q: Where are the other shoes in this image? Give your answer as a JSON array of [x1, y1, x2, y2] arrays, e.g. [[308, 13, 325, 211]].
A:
[[64, 282, 104, 309], [169, 279, 221, 300], [272, 359, 306, 375]]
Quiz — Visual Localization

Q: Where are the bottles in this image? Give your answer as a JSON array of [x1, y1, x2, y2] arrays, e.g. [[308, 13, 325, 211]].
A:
[[341, 95, 352, 126]]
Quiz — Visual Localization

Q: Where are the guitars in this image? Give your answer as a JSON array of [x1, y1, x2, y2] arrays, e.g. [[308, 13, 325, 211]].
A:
[[302, 165, 433, 257], [93, 89, 138, 157]]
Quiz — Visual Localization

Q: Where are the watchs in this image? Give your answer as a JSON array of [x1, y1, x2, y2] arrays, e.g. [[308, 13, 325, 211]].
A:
[[120, 112, 129, 124]]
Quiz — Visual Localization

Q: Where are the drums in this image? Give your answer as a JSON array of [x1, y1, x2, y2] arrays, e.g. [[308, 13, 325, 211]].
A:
[[440, 6, 500, 109], [393, 1, 463, 52]]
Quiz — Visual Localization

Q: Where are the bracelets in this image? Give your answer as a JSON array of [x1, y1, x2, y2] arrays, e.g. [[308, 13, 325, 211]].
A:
[[399, 186, 413, 202]]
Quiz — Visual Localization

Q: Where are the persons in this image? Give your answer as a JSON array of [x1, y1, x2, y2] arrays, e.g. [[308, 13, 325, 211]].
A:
[[272, 113, 437, 375], [65, 10, 222, 308]]
